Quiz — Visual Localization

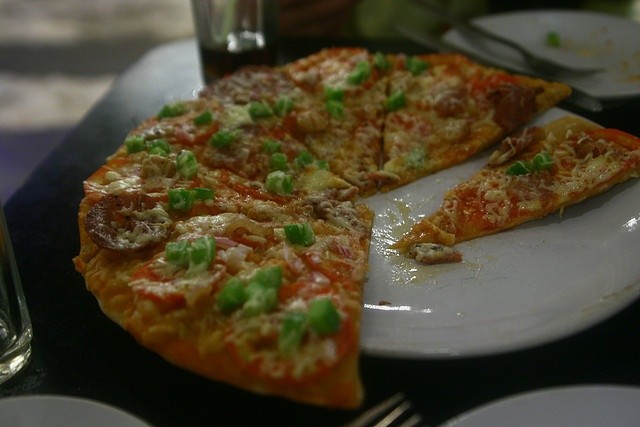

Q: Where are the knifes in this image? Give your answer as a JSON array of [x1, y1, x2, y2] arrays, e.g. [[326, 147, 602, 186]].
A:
[[394, 19, 604, 113]]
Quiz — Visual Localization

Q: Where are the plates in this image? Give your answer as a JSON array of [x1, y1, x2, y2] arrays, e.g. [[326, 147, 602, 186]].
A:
[[442, 9, 640, 102], [353, 104, 640, 362], [438, 382, 640, 427], [0, 393, 149, 427]]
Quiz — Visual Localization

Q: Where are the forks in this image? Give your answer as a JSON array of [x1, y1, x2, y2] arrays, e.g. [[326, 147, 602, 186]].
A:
[[415, 1, 608, 82], [346, 391, 424, 427]]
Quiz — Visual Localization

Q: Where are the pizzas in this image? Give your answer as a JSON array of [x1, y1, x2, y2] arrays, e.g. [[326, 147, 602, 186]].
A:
[[72, 45, 572, 409], [388, 113, 636, 263]]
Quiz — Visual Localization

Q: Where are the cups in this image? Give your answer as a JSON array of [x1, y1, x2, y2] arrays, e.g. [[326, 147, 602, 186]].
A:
[[191, 0, 274, 80], [0, 200, 35, 385]]
